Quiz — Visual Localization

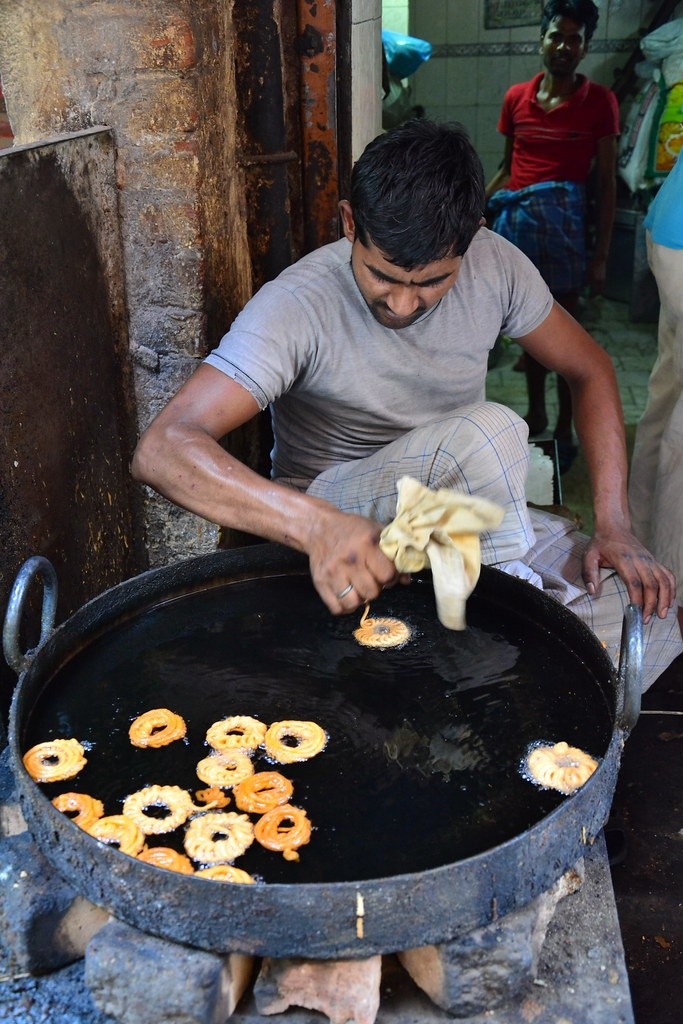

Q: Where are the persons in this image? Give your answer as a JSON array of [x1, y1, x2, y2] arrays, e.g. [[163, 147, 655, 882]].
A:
[[129, 120, 683, 695], [484, 0, 620, 438], [628, 146, 683, 608]]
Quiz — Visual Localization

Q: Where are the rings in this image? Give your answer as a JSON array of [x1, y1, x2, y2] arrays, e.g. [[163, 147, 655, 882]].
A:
[[337, 584, 353, 598]]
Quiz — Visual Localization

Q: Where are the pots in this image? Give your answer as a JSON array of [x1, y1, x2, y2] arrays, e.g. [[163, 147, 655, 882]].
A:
[[5, 558, 643, 961]]
